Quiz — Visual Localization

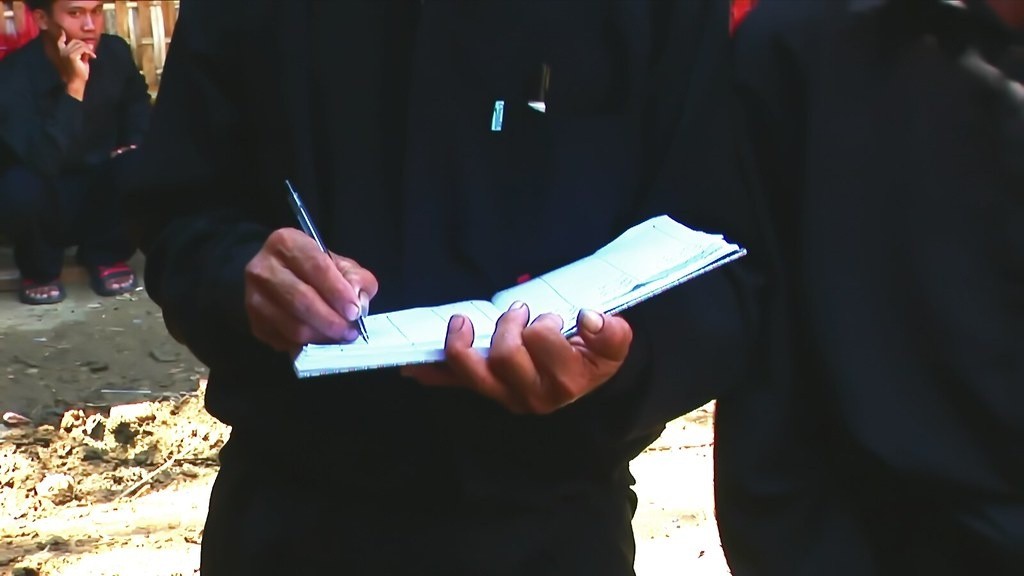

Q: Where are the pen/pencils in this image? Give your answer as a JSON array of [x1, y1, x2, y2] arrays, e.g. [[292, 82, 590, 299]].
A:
[[283, 180, 369, 343]]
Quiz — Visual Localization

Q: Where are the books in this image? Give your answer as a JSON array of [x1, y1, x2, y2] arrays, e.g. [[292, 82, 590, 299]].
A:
[[293, 213, 748, 380]]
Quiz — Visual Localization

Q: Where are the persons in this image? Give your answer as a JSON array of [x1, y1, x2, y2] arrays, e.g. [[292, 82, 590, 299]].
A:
[[0, 0, 152, 305], [135, 0, 751, 576], [712, 0, 1024, 576]]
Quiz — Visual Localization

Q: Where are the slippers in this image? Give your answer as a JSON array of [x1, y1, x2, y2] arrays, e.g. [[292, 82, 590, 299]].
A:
[[91, 260, 139, 296], [19, 279, 66, 304]]
